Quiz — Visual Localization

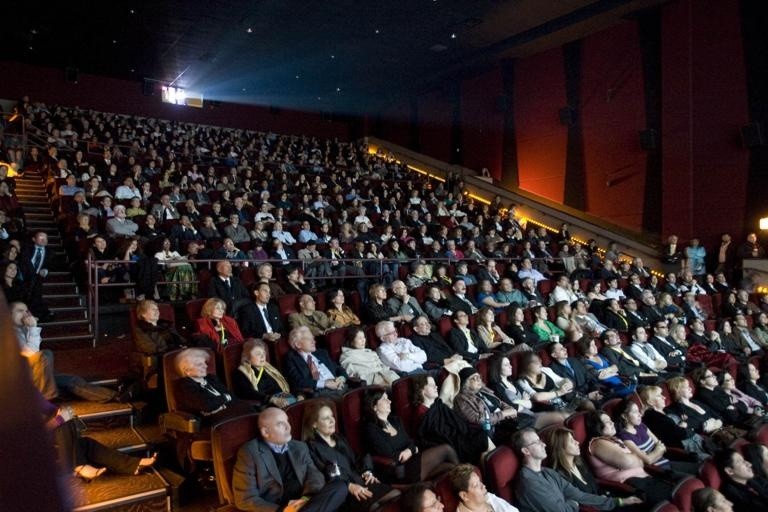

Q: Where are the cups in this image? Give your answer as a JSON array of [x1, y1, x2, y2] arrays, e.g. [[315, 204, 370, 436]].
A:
[[552, 334, 560, 343]]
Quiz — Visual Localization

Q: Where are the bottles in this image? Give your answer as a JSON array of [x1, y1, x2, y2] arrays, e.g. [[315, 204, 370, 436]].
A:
[[481, 409, 492, 435]]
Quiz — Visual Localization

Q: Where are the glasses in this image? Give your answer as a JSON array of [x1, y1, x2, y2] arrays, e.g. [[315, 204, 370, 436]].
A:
[[468, 374, 482, 383], [658, 325, 669, 329]]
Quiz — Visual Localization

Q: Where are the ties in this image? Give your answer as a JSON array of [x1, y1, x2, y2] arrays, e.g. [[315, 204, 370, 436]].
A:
[[34, 247, 42, 272], [307, 354, 320, 382], [163, 207, 168, 220]]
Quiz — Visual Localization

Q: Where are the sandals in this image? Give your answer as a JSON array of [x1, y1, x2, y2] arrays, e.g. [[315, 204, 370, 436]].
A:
[[74, 464, 107, 484], [134, 449, 161, 476]]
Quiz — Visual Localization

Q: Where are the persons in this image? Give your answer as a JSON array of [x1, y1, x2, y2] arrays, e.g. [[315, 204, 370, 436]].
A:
[[360, 157, 767, 512], [1, 95, 400, 512]]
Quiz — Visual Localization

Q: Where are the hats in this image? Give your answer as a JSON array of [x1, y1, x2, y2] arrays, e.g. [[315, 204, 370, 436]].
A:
[[458, 366, 478, 386]]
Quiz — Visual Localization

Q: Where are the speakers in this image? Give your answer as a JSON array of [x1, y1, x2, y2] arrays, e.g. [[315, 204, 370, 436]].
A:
[[639, 130, 657, 149], [738, 122, 766, 149], [558, 107, 574, 123], [495, 96, 510, 111]]
